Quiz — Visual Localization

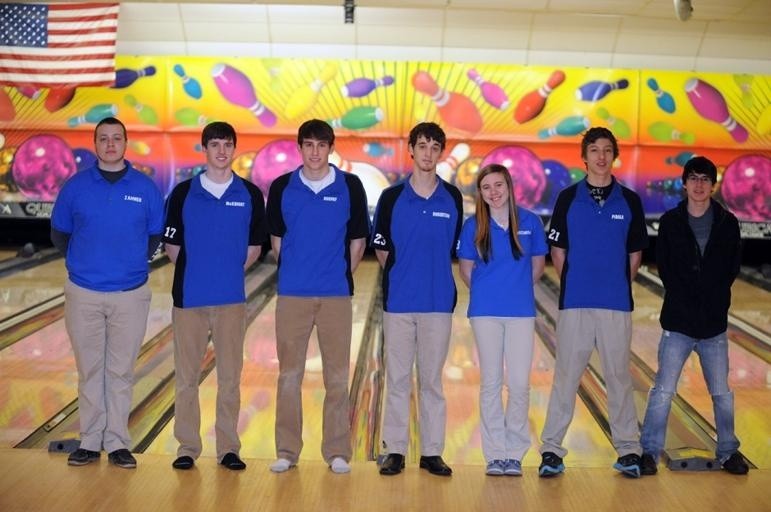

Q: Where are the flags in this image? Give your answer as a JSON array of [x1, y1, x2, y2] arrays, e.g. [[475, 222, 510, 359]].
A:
[[0, 0, 121, 90]]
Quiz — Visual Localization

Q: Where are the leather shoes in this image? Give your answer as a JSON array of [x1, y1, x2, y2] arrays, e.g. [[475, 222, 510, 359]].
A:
[[420, 455, 452, 475], [379, 452, 405, 476]]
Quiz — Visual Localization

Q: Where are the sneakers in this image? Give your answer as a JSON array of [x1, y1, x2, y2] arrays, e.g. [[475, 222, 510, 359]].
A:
[[722, 453, 749, 476], [505, 458, 523, 476], [613, 455, 641, 477], [640, 453, 656, 475], [486, 459, 504, 476], [108, 449, 137, 469], [68, 447, 101, 466], [537, 451, 565, 477]]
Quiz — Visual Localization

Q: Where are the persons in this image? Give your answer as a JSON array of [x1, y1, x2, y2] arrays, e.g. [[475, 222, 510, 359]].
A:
[[455, 163, 550, 476], [47, 116, 165, 468], [638, 154, 752, 475], [265, 119, 371, 473], [364, 119, 465, 477], [159, 121, 266, 470], [536, 125, 651, 478]]
